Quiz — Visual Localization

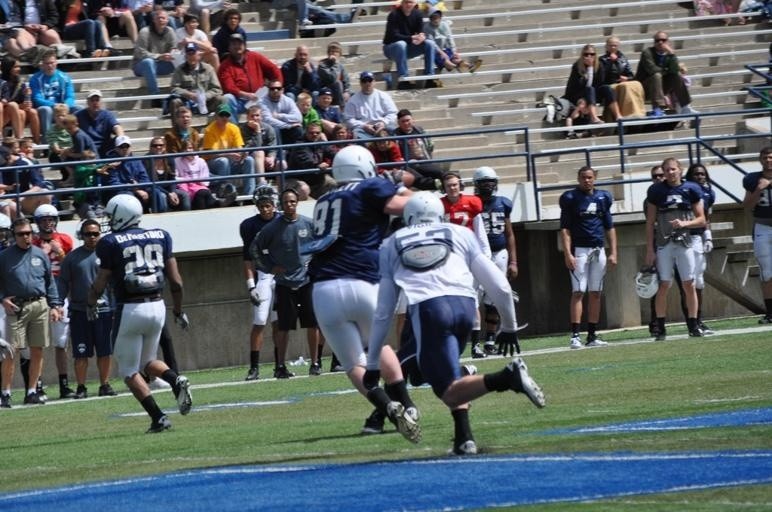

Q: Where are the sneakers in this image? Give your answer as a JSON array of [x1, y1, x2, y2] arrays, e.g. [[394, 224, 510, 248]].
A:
[[471, 348, 487, 358], [362, 401, 423, 445], [146, 415, 172, 434], [505, 358, 545, 410], [447, 58, 482, 73], [759, 313, 769, 324], [246, 359, 344, 381], [174, 375, 193, 416], [1, 384, 119, 409], [584, 339, 608, 347], [681, 107, 699, 114], [570, 338, 585, 349], [446, 440, 477, 456], [102, 49, 110, 57], [649, 320, 713, 340], [484, 341, 501, 355], [91, 50, 101, 57]]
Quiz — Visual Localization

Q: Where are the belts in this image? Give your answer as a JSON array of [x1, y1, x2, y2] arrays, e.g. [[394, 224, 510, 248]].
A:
[[13, 297, 41, 302]]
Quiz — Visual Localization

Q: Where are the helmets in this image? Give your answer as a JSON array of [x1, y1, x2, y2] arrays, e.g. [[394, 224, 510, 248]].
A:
[[402, 190, 444, 225], [634, 272, 660, 299], [319, 86, 334, 96], [0, 212, 13, 231], [474, 167, 499, 194], [103, 194, 144, 232], [34, 203, 59, 233], [333, 145, 378, 185]]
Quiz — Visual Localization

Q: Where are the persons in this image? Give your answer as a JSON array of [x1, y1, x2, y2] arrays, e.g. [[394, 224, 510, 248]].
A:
[[362, 191, 548, 454], [1, 1, 519, 406], [566, 30, 699, 123], [87, 193, 194, 435], [305, 143, 422, 435], [740, 147, 770, 324], [693, 1, 770, 26], [641, 157, 716, 341], [558, 167, 617, 349]]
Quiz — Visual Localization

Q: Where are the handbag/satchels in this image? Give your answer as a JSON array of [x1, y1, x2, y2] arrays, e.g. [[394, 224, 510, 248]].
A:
[[536, 96, 575, 124]]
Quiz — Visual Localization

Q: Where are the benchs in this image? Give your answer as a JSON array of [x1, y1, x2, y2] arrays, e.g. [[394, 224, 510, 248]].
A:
[[1, 0, 771, 221]]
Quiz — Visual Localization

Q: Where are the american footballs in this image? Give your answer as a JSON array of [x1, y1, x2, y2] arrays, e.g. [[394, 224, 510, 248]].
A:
[[383, 170, 416, 188]]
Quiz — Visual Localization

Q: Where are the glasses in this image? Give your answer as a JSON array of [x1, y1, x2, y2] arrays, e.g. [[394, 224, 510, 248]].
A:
[[362, 78, 373, 83], [269, 86, 283, 92], [652, 174, 663, 179], [118, 144, 130, 149], [220, 112, 231, 118], [655, 38, 668, 43], [82, 231, 100, 237], [582, 52, 597, 57], [639, 266, 657, 275], [14, 230, 32, 236], [150, 144, 165, 147]]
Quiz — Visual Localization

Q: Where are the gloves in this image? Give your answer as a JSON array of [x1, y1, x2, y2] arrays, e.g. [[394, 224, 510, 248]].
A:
[[378, 168, 408, 195], [249, 288, 262, 307], [704, 240, 714, 254], [86, 303, 101, 322], [493, 324, 528, 357], [174, 311, 189, 330]]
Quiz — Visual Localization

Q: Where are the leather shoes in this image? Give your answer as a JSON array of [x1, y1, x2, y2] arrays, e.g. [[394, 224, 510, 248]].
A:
[[107, 47, 123, 56]]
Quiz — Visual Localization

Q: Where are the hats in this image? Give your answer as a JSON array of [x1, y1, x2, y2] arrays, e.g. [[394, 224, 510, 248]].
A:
[[230, 34, 244, 42], [87, 89, 103, 99], [216, 104, 231, 117], [186, 42, 198, 51], [115, 135, 132, 147], [360, 71, 375, 80], [327, 43, 342, 55]]
